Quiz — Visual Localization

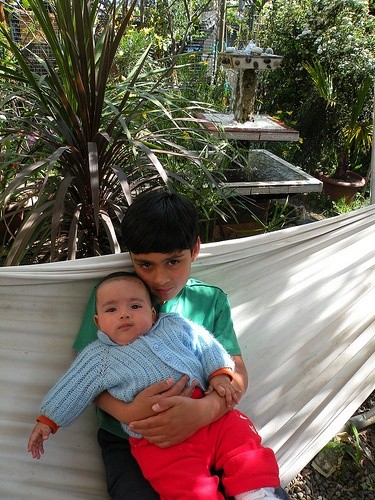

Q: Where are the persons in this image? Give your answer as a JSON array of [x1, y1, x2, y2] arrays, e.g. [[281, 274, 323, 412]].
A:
[[27, 270, 283, 500], [73, 190, 291, 500]]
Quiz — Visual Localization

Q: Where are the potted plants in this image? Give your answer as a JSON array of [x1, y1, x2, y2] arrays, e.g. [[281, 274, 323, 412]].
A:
[[302, 58, 374, 206]]
